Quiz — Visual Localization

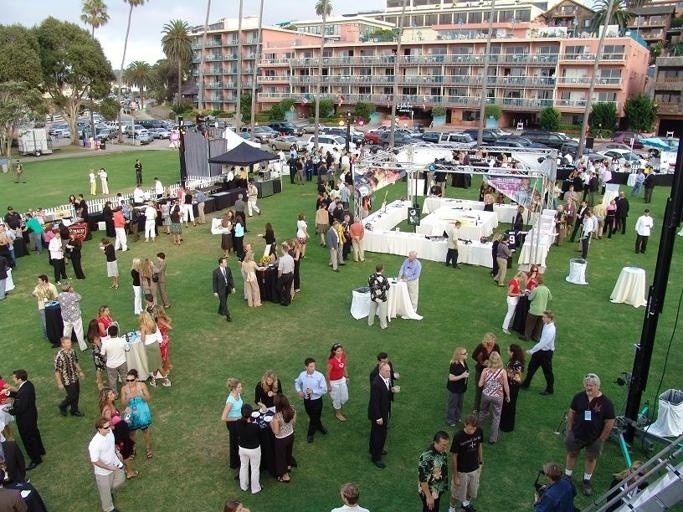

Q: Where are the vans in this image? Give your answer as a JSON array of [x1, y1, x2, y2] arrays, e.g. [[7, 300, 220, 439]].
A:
[[610, 131, 647, 148]]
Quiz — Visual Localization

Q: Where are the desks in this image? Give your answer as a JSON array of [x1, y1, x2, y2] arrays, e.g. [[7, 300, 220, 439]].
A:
[[421, 194, 538, 226], [415, 205, 499, 242], [356, 200, 494, 269], [638, 165, 676, 186], [246, 408, 276, 475], [256, 262, 280, 302], [376, 277, 424, 323], [517, 208, 559, 274], [5, 266, 15, 291], [119, 332, 152, 383], [86, 175, 283, 232], [1, 214, 77, 258], [608, 266, 648, 309], [44, 299, 78, 348]]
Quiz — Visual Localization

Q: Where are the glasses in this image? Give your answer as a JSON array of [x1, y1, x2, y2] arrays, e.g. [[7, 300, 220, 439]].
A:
[[102, 424, 111, 430], [126, 378, 137, 383], [460, 352, 466, 356], [531, 270, 538, 273]]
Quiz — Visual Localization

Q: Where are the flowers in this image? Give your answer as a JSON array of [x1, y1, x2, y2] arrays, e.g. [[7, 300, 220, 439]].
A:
[[258, 255, 272, 267]]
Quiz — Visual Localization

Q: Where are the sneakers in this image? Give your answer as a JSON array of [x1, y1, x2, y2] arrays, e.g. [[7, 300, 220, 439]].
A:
[[320, 426, 328, 436], [581, 474, 594, 495], [58, 403, 69, 417], [69, 410, 86, 419], [306, 432, 315, 444], [335, 414, 347, 422]]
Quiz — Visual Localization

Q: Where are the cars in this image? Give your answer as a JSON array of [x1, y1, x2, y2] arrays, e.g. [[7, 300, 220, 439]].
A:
[[210, 126, 279, 146], [595, 147, 650, 171], [367, 125, 422, 151], [48, 114, 119, 141], [298, 123, 326, 135], [269, 136, 305, 151], [118, 119, 192, 144], [522, 130, 571, 149]]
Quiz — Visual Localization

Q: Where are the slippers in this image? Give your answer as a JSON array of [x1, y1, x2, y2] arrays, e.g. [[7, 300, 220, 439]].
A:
[[278, 474, 291, 484]]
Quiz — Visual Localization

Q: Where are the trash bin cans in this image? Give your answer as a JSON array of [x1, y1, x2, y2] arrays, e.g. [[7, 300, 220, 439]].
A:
[[644, 389, 683, 438], [350, 287, 372, 320], [565, 258, 589, 285]]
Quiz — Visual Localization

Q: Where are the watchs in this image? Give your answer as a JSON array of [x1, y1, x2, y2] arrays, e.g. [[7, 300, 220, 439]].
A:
[[77, 369, 82, 373], [599, 436, 607, 442], [416, 431, 450, 512]]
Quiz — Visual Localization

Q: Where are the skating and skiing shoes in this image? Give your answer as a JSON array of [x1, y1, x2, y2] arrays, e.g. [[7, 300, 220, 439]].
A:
[[460, 501, 479, 512]]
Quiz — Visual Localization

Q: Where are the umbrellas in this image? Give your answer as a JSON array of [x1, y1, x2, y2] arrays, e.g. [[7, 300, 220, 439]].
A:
[[639, 138, 669, 151]]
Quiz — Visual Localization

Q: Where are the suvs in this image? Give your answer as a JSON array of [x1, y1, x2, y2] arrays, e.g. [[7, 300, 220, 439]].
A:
[[270, 122, 293, 133], [465, 127, 513, 143], [421, 132, 488, 149], [305, 134, 358, 155], [329, 127, 366, 146], [494, 136, 550, 148]]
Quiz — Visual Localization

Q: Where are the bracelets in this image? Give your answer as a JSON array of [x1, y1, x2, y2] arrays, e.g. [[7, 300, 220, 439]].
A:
[[346, 376, 350, 378]]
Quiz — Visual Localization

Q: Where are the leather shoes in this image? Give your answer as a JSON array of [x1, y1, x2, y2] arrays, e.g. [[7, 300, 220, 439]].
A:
[[25, 460, 42, 470], [226, 316, 231, 322], [380, 448, 388, 456], [538, 389, 554, 396], [374, 458, 386, 469], [125, 470, 140, 481]]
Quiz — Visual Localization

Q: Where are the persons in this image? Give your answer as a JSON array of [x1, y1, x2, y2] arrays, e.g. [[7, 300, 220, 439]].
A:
[[1, 369, 46, 512], [488, 233, 502, 278], [96, 304, 119, 337], [134, 159, 143, 184], [32, 274, 59, 340], [431, 157, 447, 198], [53, 337, 87, 417], [632, 168, 645, 198], [0, 192, 43, 260], [87, 319, 106, 391], [331, 482, 370, 512], [397, 250, 422, 312], [121, 369, 153, 460], [217, 194, 246, 258], [642, 167, 656, 203], [471, 332, 500, 415], [268, 242, 280, 303], [246, 181, 261, 217], [232, 404, 265, 496], [263, 222, 274, 256], [501, 271, 525, 335], [278, 144, 362, 181], [479, 180, 497, 211], [605, 460, 649, 512], [226, 167, 236, 188], [554, 153, 629, 259], [104, 178, 207, 250], [644, 165, 651, 178], [478, 462, 484, 464], [278, 238, 301, 306], [238, 244, 252, 301], [514, 205, 524, 251], [239, 168, 248, 188], [315, 181, 371, 271], [255, 369, 282, 412], [635, 208, 653, 255], [525, 265, 542, 291], [88, 417, 127, 512], [449, 414, 484, 512], [520, 310, 557, 395], [152, 304, 173, 376], [223, 498, 251, 512], [369, 352, 400, 402], [212, 257, 236, 323], [242, 252, 267, 308], [43, 224, 86, 285], [478, 351, 511, 445], [269, 394, 298, 483], [102, 238, 120, 289], [446, 346, 469, 428], [445, 221, 463, 268], [101, 325, 131, 401], [89, 167, 110, 195], [294, 357, 327, 444], [368, 263, 390, 330], [138, 311, 171, 388], [452, 150, 471, 165], [130, 258, 143, 315], [367, 361, 400, 468], [500, 344, 526, 432], [494, 237, 509, 286], [69, 194, 92, 241], [326, 342, 350, 422], [564, 373, 616, 497], [58, 281, 89, 352], [519, 275, 552, 342], [151, 252, 171, 309], [221, 377, 244, 469], [97, 388, 138, 480], [532, 462, 581, 512], [492, 152, 530, 175], [296, 212, 310, 258]]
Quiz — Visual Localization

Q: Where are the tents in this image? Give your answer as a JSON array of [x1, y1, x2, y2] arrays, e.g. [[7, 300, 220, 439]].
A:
[[207, 142, 281, 178]]
